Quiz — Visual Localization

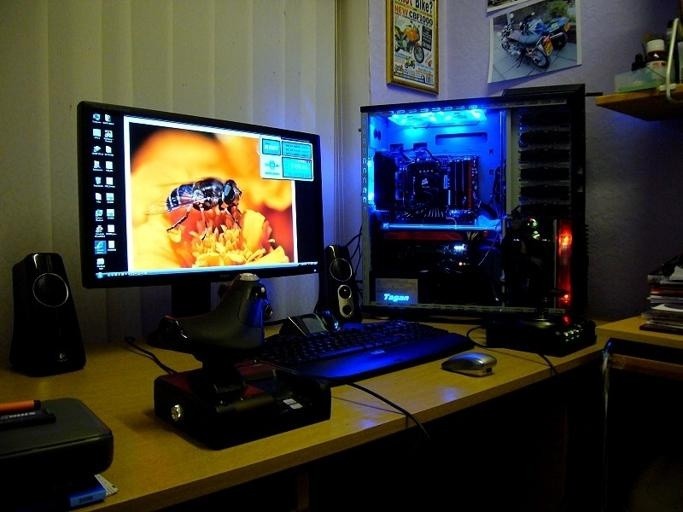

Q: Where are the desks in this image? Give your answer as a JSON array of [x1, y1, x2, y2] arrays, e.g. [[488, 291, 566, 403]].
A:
[[0, 317, 608, 512]]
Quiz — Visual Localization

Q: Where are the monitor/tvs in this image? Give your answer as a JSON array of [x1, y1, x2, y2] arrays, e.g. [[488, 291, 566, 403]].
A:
[[77, 100, 323, 318]]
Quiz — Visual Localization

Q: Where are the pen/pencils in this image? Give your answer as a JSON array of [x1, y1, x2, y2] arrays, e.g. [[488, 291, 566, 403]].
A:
[[0, 399, 40, 412]]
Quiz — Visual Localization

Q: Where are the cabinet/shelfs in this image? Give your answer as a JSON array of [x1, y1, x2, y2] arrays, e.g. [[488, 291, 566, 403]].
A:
[[594, 84, 683, 379]]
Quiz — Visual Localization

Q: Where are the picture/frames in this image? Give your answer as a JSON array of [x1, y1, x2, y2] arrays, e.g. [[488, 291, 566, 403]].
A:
[[386, 0, 439, 96]]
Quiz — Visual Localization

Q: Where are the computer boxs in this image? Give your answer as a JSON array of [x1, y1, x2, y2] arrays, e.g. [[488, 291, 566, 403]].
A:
[[359, 83, 588, 325]]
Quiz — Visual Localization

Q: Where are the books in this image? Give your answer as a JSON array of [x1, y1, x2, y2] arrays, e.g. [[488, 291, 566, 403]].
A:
[[638, 253, 682, 335]]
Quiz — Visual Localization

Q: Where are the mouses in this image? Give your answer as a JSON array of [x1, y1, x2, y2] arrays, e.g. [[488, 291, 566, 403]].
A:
[[441, 351, 498, 376]]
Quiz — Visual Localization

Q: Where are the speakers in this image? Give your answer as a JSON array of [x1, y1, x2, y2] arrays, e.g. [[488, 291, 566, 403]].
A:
[[320, 244, 360, 321], [14, 253, 86, 378]]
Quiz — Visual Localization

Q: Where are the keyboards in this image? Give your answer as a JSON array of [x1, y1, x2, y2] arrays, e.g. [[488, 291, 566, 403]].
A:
[[260, 320, 472, 380]]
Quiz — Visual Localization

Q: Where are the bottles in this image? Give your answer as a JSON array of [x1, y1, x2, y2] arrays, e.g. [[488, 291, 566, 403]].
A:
[[646, 39, 668, 85]]
